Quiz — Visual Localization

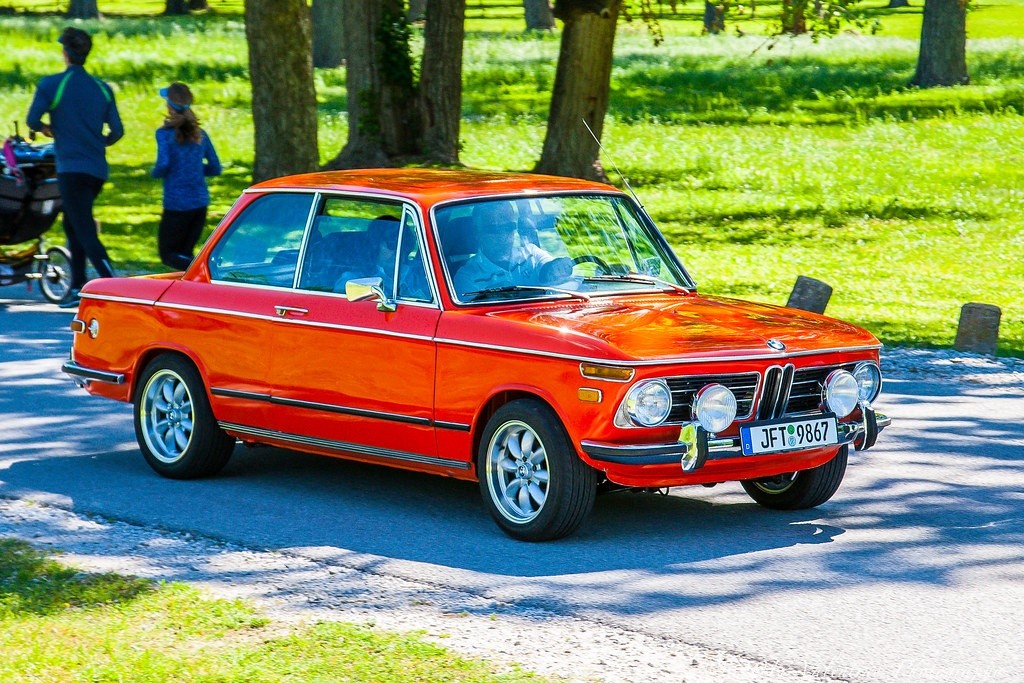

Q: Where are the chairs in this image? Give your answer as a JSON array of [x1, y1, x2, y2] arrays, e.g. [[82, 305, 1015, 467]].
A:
[[442, 217, 477, 281], [308, 230, 367, 290]]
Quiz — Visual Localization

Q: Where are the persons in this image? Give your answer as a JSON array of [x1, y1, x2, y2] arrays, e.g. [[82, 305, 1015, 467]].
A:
[[25, 25, 125, 308], [451, 197, 575, 304], [148, 81, 223, 273], [333, 212, 433, 306]]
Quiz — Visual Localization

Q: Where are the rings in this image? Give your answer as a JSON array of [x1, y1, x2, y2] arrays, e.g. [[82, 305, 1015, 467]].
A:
[[565, 265, 570, 269]]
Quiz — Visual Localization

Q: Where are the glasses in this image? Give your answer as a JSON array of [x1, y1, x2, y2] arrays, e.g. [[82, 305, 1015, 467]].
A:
[[479, 222, 517, 235], [385, 240, 409, 254]]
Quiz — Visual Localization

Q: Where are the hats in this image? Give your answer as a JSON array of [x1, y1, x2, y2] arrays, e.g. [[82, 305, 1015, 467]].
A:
[[58, 27, 91, 54], [160, 86, 190, 111]]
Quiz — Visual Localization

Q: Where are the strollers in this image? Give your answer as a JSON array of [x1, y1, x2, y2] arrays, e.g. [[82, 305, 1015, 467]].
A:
[[0, 133, 74, 305]]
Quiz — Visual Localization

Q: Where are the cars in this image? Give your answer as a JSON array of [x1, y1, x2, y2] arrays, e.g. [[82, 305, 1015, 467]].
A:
[[62, 167, 893, 545]]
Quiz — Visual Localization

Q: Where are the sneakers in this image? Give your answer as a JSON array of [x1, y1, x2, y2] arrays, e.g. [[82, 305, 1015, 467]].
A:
[[60, 288, 84, 308]]
